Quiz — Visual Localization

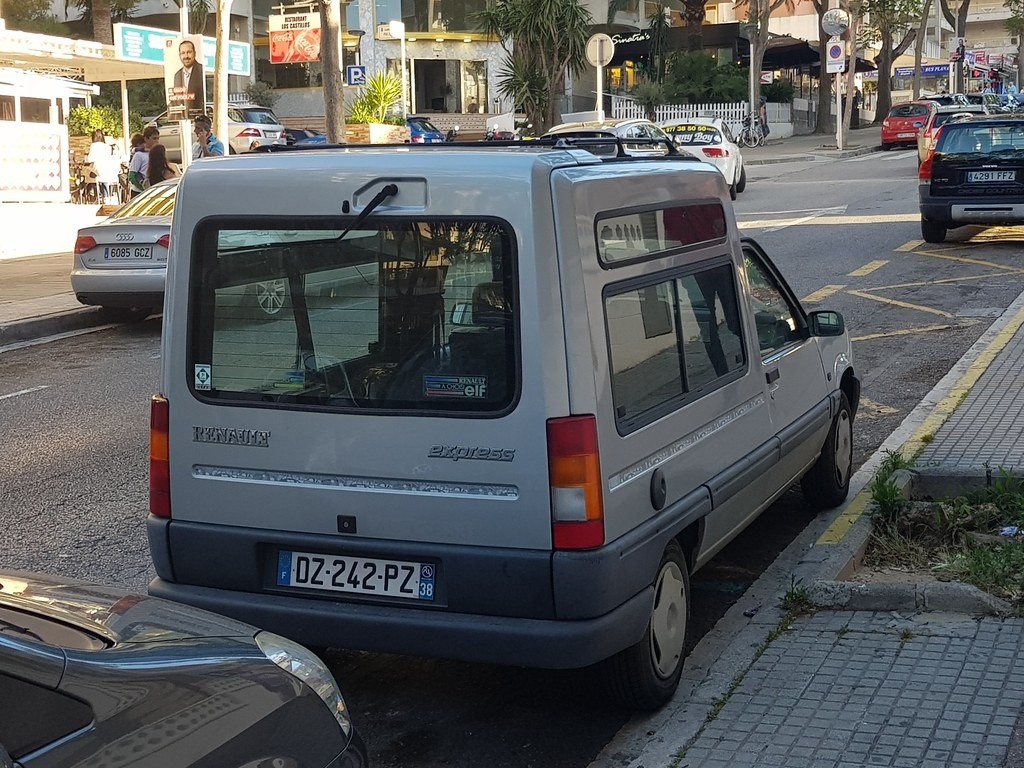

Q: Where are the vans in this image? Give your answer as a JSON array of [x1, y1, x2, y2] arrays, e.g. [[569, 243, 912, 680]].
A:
[[140, 147, 863, 716]]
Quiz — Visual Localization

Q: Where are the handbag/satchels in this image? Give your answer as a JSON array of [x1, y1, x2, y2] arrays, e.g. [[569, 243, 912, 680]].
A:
[[762, 124, 770, 138]]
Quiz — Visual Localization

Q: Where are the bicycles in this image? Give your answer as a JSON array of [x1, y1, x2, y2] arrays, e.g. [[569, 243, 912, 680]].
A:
[[735, 110, 765, 148]]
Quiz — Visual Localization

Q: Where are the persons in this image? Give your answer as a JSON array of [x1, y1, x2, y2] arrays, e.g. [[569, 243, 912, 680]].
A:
[[249, 141, 261, 150], [87, 127, 108, 204], [663, 171, 743, 376], [191, 115, 224, 159], [126, 127, 179, 201], [171, 40, 205, 120], [982, 82, 995, 93], [1008, 82, 1016, 94], [955, 39, 965, 62], [760, 96, 770, 138]]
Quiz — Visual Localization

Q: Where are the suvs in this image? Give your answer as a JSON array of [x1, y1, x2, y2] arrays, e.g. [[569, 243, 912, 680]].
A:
[[544, 116, 698, 161]]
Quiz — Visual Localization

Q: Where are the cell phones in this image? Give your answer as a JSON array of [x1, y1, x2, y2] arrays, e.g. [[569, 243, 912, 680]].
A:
[[204, 126, 210, 132]]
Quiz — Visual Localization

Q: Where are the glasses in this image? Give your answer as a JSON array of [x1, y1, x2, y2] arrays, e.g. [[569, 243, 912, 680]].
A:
[[195, 115, 210, 123]]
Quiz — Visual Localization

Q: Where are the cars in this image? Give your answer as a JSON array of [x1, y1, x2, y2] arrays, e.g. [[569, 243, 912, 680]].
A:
[[69, 176, 395, 323], [880, 93, 1024, 150], [0, 567, 370, 767], [494, 129, 515, 141], [918, 113, 1024, 245], [134, 102, 289, 165], [288, 115, 448, 146], [652, 117, 749, 200], [914, 102, 991, 172], [282, 126, 321, 145]]
